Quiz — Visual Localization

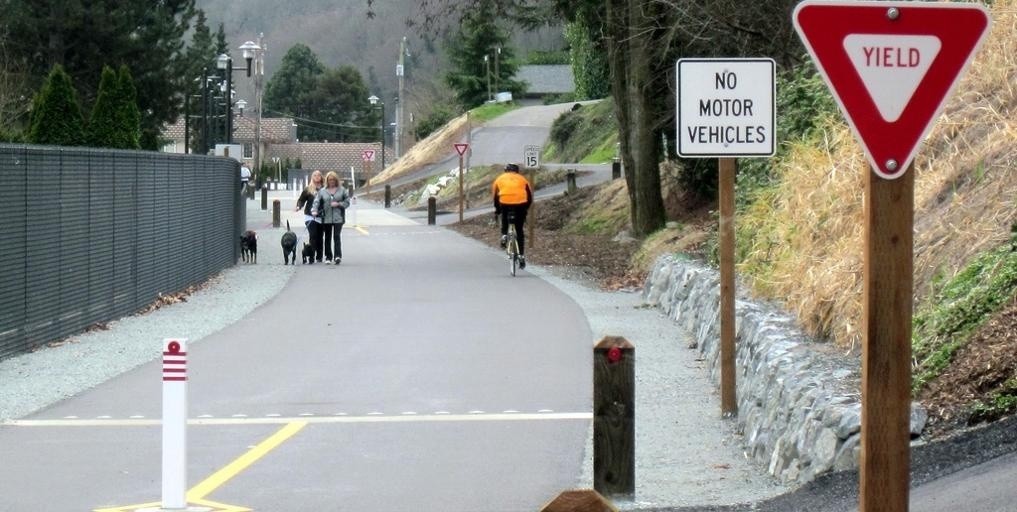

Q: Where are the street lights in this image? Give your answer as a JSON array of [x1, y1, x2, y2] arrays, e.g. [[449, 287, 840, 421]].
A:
[[483, 54, 491, 101], [184, 39, 263, 156], [494, 44, 502, 92], [367, 94, 389, 170]]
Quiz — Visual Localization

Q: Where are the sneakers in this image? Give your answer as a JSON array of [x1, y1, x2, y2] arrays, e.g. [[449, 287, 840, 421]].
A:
[[335, 257, 341, 264], [519, 255, 525, 269], [501, 235, 507, 249], [325, 260, 331, 264]]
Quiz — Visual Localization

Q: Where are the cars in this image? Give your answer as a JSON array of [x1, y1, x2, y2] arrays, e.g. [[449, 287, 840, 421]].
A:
[[240, 166, 252, 191]]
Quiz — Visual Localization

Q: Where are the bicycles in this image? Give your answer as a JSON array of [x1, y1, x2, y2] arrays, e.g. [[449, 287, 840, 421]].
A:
[[496, 207, 524, 277]]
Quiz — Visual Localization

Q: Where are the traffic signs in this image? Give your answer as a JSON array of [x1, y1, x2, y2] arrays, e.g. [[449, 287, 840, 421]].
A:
[[524, 145, 539, 169]]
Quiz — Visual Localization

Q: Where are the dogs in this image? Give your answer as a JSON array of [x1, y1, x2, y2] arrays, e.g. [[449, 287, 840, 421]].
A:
[[302, 242, 314, 264], [281, 219, 297, 265], [240, 230, 256, 264]]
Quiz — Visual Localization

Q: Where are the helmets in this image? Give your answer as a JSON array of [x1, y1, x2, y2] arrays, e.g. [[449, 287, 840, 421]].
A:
[[504, 164, 519, 173]]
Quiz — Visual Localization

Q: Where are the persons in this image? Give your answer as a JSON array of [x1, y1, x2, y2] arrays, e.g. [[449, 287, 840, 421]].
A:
[[493, 164, 533, 270], [240, 162, 251, 189], [311, 171, 350, 264], [295, 170, 325, 263]]
